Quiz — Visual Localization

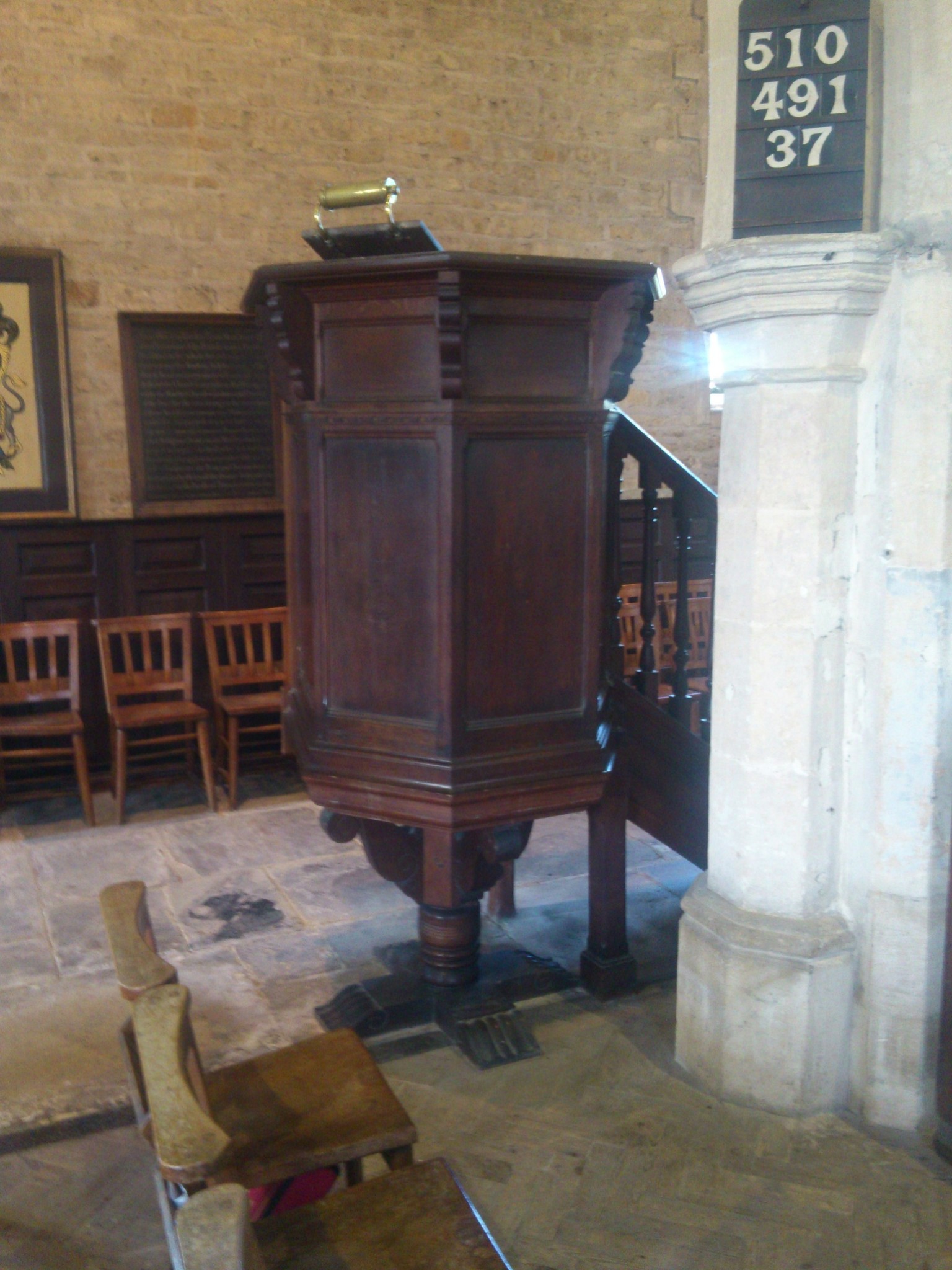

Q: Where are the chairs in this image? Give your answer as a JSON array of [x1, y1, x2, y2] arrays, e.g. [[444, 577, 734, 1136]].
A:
[[173, 1201, 270, 1270], [90, 612, 216, 823], [199, 604, 292, 806], [96, 876, 418, 1270], [0, 616, 98, 826], [127, 985, 515, 1270], [618, 583, 712, 740]]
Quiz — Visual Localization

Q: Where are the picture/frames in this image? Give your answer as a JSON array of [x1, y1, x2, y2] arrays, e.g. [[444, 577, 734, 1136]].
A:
[[0, 248, 77, 524]]
